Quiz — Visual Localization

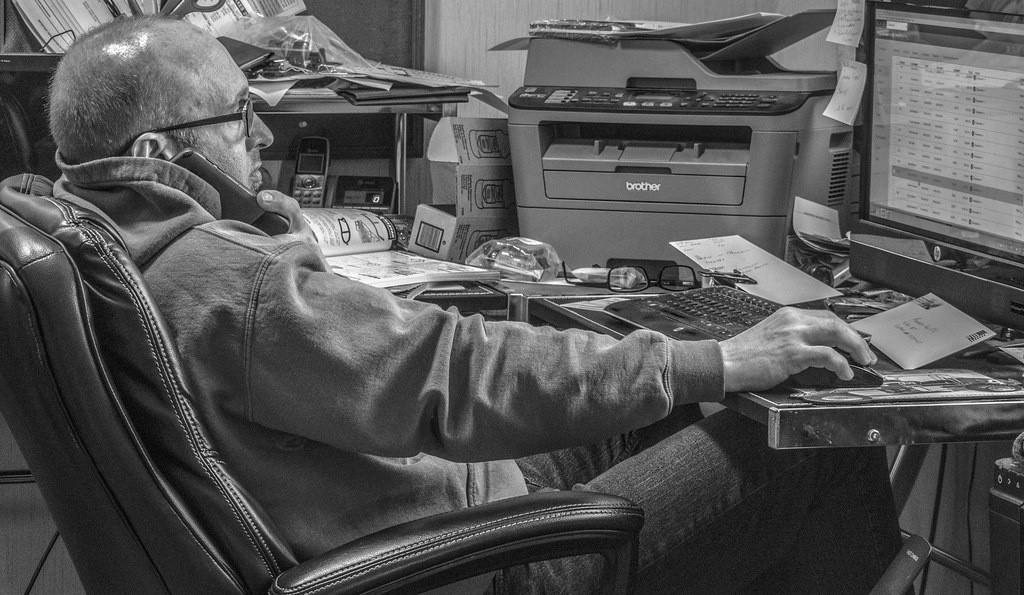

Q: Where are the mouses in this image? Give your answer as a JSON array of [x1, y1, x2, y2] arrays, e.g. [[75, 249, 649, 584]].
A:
[[785, 346, 884, 390]]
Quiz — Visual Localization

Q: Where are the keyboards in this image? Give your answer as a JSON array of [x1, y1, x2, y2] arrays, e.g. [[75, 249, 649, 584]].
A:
[[603, 286, 786, 342]]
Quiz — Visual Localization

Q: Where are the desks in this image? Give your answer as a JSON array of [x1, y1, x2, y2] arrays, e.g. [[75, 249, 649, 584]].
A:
[[528, 295, 1024, 595]]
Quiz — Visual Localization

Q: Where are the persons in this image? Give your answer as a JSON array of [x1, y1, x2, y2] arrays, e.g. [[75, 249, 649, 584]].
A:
[[48, 19, 915, 595]]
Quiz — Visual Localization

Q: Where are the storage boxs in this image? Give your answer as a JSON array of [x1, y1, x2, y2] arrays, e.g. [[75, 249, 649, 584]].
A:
[[410, 118, 517, 260]]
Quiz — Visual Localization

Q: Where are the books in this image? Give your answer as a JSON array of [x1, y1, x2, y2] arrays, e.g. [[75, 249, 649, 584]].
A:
[[391, 283, 432, 299], [298, 207, 500, 288]]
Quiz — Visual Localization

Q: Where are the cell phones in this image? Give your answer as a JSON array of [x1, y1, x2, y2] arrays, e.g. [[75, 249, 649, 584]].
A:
[[290, 136, 330, 208], [168, 148, 291, 237]]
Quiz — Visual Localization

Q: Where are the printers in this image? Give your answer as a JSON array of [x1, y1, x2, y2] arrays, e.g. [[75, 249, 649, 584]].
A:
[[510, 8, 862, 284]]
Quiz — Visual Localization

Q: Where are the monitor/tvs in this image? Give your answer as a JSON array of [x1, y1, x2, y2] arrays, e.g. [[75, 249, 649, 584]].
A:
[[849, 3, 1024, 356]]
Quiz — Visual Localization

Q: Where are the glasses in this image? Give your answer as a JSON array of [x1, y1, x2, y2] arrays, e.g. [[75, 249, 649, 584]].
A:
[[562, 260, 698, 293], [117, 97, 253, 158]]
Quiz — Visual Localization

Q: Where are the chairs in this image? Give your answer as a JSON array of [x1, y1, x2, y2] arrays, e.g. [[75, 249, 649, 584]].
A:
[[0, 174, 644, 595]]
